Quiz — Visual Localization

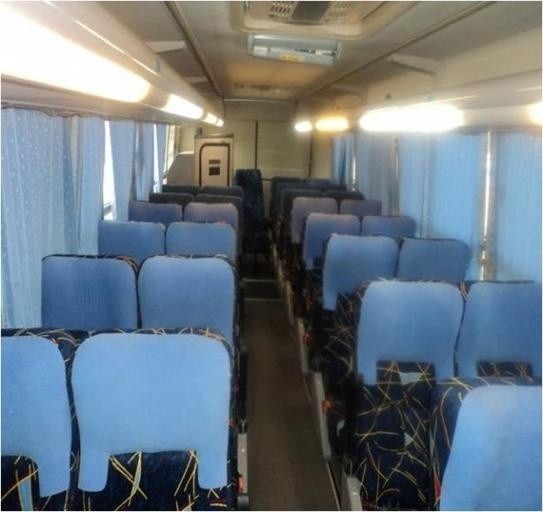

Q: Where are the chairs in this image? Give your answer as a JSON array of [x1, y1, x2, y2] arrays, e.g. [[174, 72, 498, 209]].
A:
[[70, 326, 247, 510], [40, 185, 246, 373], [233, 167, 271, 268], [0, 324, 76, 511], [270, 178, 539, 512]]
